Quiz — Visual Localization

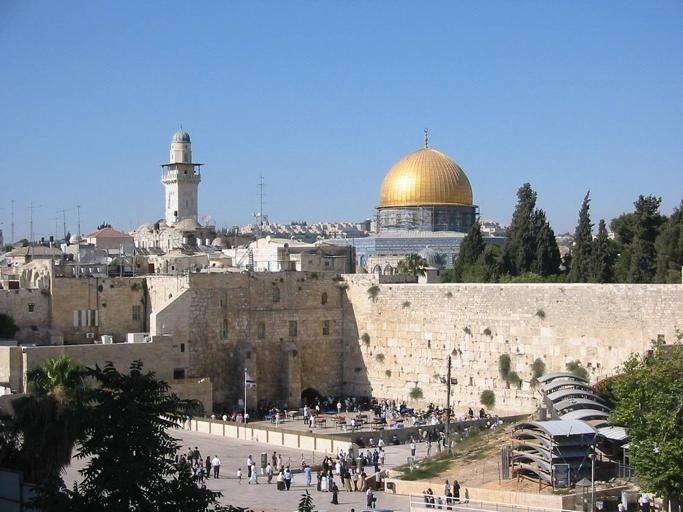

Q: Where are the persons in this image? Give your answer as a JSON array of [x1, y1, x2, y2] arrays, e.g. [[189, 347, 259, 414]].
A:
[[393, 426, 458, 457], [422, 479, 461, 511], [181, 445, 220, 484], [237, 437, 386, 510], [302, 399, 485, 431], [233, 402, 288, 427]]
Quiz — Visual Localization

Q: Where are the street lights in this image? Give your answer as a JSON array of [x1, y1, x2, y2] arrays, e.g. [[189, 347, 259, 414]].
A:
[[440, 356, 457, 448]]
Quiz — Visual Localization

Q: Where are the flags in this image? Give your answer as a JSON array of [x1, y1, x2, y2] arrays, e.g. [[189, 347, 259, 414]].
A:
[[245, 372, 256, 389]]
